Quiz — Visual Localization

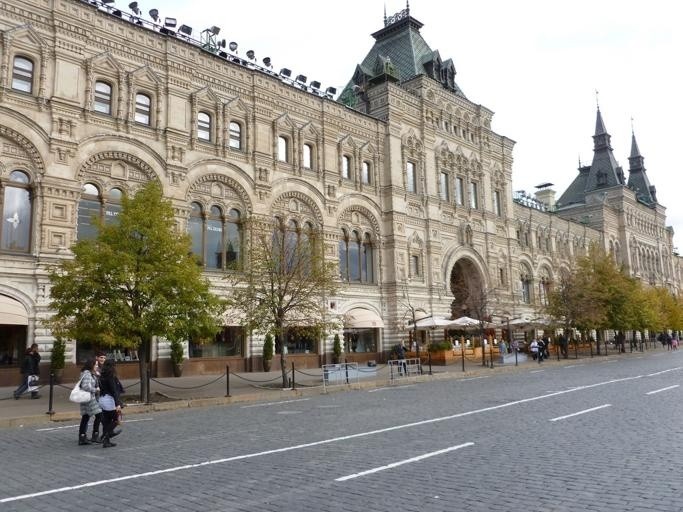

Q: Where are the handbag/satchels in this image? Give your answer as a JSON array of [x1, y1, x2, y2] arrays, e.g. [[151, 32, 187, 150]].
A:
[[27, 375, 40, 392], [108, 410, 123, 438], [68, 373, 92, 403]]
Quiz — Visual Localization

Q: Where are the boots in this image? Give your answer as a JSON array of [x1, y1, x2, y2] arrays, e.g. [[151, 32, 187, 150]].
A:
[[102, 436, 117, 448], [90, 431, 106, 443], [77, 433, 92, 445]]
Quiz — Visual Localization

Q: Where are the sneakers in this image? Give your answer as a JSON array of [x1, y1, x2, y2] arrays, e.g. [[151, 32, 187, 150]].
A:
[[13, 390, 20, 400], [30, 395, 40, 399]]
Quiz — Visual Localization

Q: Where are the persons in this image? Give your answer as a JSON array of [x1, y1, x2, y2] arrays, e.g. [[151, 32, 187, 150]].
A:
[[95, 350, 122, 440], [12, 348, 40, 400], [394, 339, 410, 376], [498, 331, 680, 362], [98, 357, 125, 447], [13, 343, 40, 395], [75, 357, 102, 445]]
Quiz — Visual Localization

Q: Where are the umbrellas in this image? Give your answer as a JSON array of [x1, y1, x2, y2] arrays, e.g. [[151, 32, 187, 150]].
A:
[[447, 316, 496, 370], [403, 316, 460, 373], [492, 310, 581, 331]]
